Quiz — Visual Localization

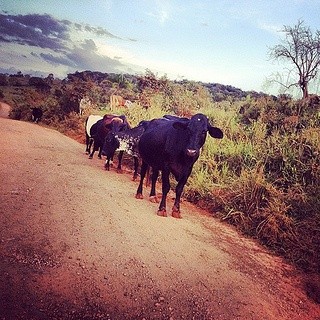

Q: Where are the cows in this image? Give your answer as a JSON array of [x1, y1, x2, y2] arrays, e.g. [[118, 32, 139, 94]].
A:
[[103, 120, 151, 188], [135, 114, 223, 219], [85, 114, 131, 168]]
